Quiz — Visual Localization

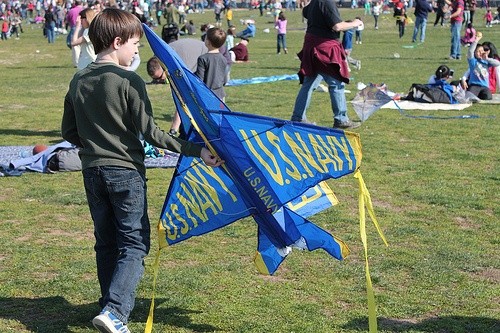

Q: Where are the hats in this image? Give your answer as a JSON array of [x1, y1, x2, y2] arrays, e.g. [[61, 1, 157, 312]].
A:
[[241, 37, 248, 42]]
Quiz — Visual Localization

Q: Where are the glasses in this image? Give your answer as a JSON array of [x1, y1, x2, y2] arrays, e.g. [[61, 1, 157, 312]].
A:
[[152, 70, 165, 80], [484, 48, 489, 51], [81, 16, 85, 18]]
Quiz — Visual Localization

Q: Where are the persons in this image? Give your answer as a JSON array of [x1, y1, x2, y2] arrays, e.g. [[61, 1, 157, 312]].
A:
[[71, 8, 97, 71], [194, 28, 230, 102], [291, 0, 363, 130], [337, 0, 500, 103], [61, 8, 224, 333], [147, 38, 208, 137], [0, 0, 310, 68]]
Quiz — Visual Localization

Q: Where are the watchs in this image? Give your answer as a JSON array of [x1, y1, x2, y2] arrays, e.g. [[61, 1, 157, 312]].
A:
[[169, 129, 177, 135]]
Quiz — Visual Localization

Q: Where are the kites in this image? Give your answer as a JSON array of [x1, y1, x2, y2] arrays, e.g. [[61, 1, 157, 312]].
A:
[[141, 21, 390, 333]]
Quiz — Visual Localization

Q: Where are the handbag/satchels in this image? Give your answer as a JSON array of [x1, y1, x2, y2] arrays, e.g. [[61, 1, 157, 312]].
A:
[[410, 83, 451, 104], [57, 148, 82, 171]]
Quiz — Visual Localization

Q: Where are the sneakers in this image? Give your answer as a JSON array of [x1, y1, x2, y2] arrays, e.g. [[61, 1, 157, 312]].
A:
[[93, 310, 132, 333], [334, 120, 360, 129]]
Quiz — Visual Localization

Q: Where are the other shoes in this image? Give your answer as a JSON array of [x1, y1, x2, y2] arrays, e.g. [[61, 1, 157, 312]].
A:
[[284, 48, 288, 54], [356, 61, 361, 70], [278, 52, 280, 54]]
